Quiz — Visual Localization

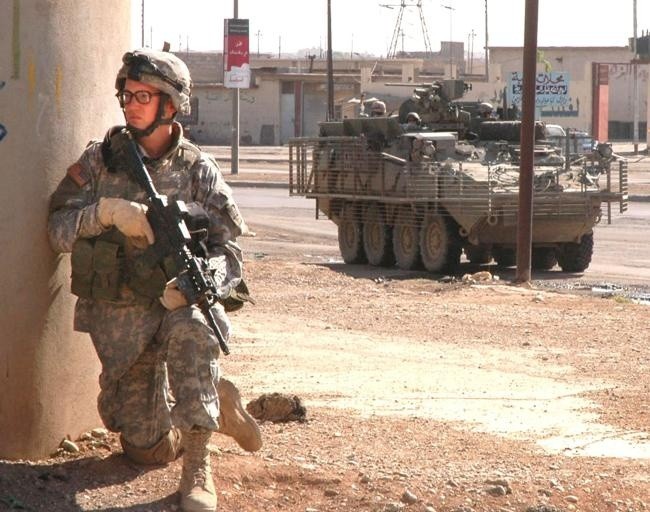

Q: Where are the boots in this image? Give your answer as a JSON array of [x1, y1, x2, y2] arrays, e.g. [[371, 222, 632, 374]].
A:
[[180, 433, 218, 511], [216, 378, 261, 453]]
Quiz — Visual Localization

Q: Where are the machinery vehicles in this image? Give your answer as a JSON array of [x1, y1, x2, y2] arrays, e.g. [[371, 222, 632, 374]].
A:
[[290, 80, 629, 273]]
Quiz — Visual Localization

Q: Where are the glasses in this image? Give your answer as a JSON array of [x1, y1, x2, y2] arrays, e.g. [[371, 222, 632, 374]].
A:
[[116, 88, 165, 105]]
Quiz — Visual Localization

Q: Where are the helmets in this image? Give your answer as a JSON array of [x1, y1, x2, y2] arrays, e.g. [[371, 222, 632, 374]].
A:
[[406, 113, 420, 125], [371, 101, 385, 113], [116, 51, 192, 114], [479, 104, 492, 113]]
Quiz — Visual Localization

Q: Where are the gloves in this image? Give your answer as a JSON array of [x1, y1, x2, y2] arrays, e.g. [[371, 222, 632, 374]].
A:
[[160, 278, 189, 310], [97, 198, 154, 246]]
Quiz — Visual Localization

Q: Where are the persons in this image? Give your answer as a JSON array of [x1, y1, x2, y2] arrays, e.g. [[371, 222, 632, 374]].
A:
[[406, 111, 421, 125], [370, 100, 387, 118], [480, 102, 495, 118], [46, 47, 264, 512]]
